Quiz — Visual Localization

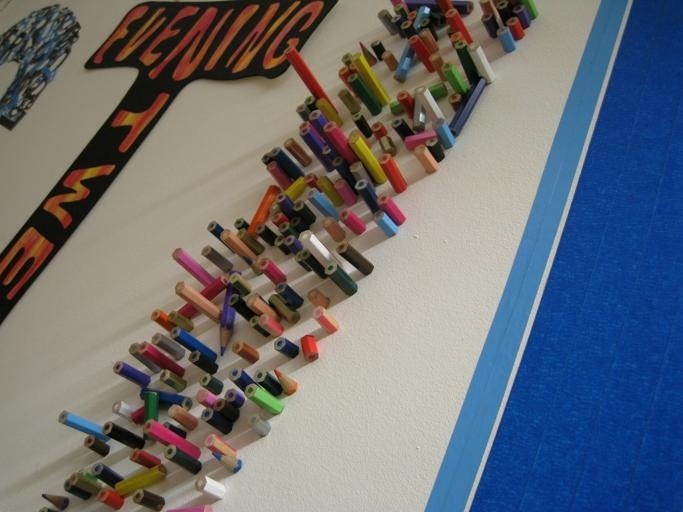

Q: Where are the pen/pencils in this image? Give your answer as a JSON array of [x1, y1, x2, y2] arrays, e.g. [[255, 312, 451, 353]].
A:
[[274, 369, 298, 395], [324, 261, 358, 296], [376, 194, 406, 226], [245, 383, 284, 415], [39, 0, 539, 512], [336, 241, 374, 275], [467, 41, 497, 84], [274, 337, 299, 358], [378, 153, 408, 193], [58, 410, 111, 442], [163, 444, 201, 475]]
